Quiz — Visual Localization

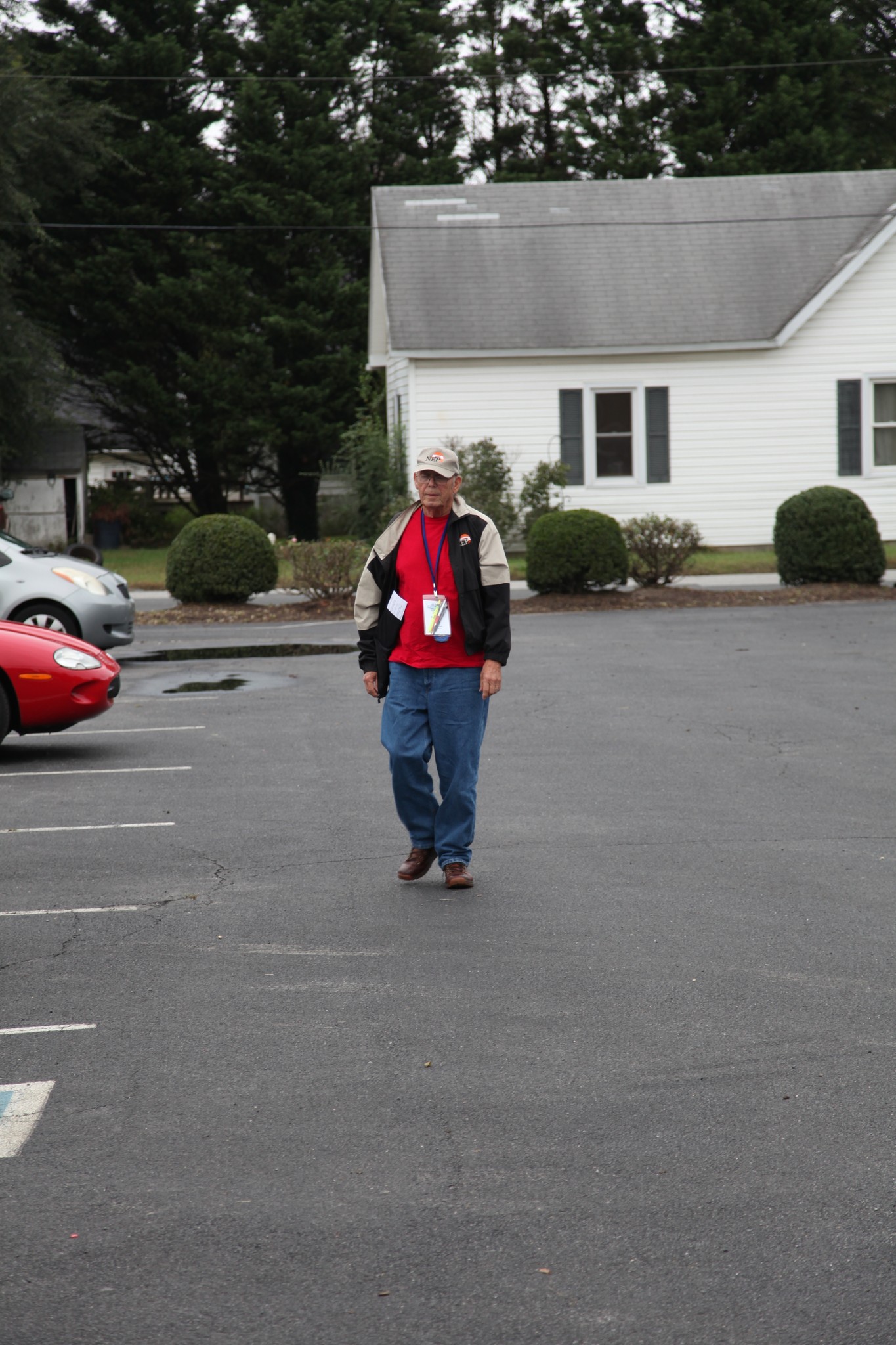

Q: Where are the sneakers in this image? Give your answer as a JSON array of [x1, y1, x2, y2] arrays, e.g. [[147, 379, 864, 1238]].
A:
[[396, 845, 438, 879], [443, 862, 474, 888]]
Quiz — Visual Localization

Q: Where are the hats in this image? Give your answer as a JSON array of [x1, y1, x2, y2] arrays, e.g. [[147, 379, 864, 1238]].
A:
[[414, 447, 459, 478]]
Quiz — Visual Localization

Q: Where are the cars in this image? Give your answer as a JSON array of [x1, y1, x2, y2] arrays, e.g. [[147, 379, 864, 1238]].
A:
[[0, 619, 123, 744], [0, 531, 137, 653]]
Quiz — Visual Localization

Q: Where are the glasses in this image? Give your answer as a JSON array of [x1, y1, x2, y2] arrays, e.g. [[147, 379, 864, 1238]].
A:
[[415, 473, 457, 485]]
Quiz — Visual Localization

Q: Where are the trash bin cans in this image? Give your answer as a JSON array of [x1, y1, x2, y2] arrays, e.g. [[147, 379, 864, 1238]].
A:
[[93, 504, 121, 549], [117, 504, 137, 544]]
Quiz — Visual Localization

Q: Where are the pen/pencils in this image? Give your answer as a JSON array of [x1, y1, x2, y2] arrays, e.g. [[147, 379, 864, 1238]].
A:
[[429, 599, 441, 631], [434, 598, 446, 628], [431, 602, 447, 635]]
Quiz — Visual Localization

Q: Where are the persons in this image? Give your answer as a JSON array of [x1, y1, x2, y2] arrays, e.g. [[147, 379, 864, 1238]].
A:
[[353, 447, 511, 888]]
